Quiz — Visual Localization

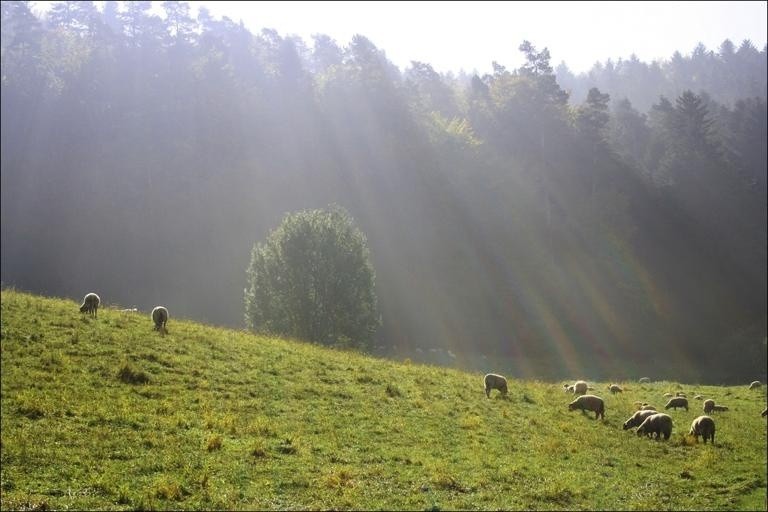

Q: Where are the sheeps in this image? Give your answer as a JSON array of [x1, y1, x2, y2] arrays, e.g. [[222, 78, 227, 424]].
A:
[[562, 381, 729, 443], [749, 379, 762, 390], [483, 373, 509, 398], [151, 305, 169, 333], [78, 292, 101, 318]]
